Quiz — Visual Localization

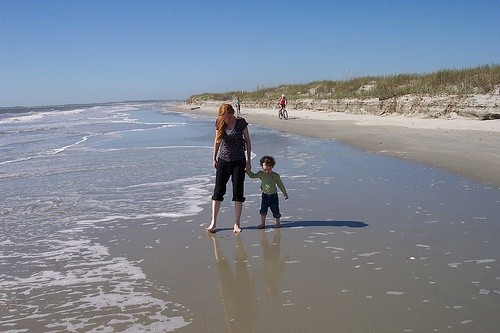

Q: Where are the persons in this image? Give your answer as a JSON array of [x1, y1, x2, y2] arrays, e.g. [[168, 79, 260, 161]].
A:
[[206, 102, 251, 233], [277, 93, 287, 115], [234, 97, 242, 114], [246, 155, 288, 227]]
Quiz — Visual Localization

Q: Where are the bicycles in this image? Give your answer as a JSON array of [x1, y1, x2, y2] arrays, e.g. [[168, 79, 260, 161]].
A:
[[278, 106, 288, 120]]
